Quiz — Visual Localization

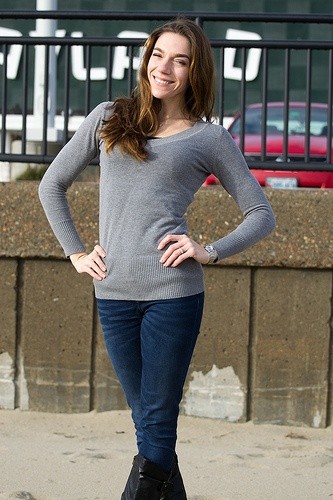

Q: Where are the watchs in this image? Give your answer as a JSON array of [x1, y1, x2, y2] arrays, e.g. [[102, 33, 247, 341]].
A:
[[203, 245, 215, 265]]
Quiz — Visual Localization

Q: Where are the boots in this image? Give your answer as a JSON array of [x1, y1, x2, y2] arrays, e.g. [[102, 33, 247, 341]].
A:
[[121, 454, 186, 500]]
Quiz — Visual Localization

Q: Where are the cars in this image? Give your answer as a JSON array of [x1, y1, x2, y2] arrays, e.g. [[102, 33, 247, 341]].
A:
[[204, 102, 333, 188]]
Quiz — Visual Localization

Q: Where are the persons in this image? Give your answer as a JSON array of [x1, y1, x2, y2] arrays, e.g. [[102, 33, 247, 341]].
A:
[[38, 19, 277, 500]]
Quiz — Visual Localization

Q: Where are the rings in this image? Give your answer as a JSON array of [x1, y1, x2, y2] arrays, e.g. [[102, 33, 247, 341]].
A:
[[179, 248, 184, 254]]
